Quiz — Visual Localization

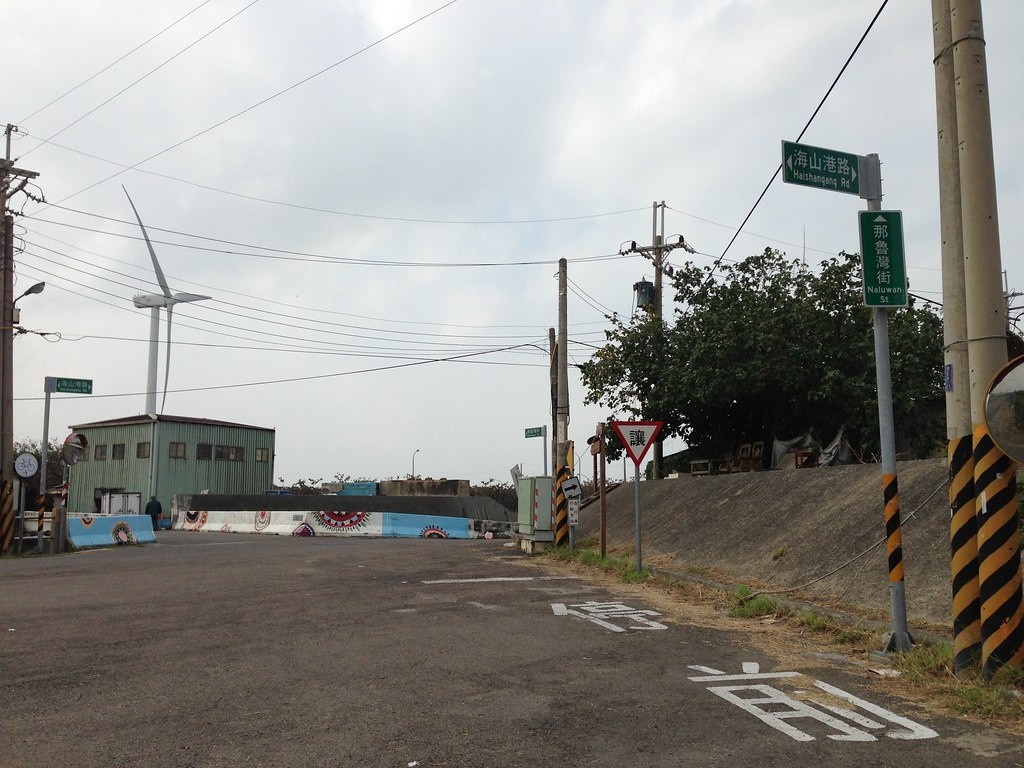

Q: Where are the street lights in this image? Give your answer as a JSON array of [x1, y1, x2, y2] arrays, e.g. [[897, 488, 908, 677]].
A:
[[412, 449, 420, 479], [0, 280, 50, 558]]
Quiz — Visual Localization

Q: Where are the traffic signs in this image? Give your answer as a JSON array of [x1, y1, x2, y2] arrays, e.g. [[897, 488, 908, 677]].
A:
[[591, 440, 602, 455], [781, 140, 861, 197], [524, 427, 541, 439], [857, 208, 910, 309], [56, 377, 94, 395]]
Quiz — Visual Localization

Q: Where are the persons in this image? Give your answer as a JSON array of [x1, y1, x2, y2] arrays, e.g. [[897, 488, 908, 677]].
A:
[[145, 496, 162, 531]]
